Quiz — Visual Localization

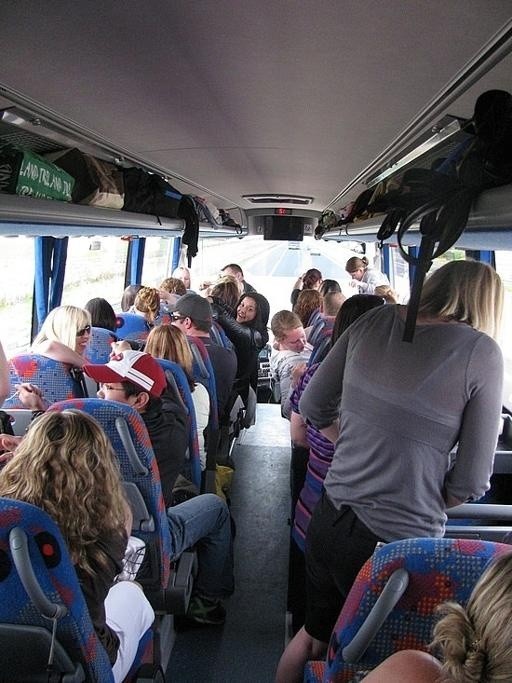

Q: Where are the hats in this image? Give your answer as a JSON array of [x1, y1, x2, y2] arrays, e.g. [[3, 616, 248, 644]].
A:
[[80, 349, 166, 399], [161, 292, 213, 322]]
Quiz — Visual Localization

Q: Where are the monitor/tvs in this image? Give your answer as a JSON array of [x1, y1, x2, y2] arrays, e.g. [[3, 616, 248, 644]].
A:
[[264, 215, 305, 241]]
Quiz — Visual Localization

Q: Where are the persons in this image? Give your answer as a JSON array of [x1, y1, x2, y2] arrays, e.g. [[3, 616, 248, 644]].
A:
[[206, 292, 271, 425], [298, 293, 346, 345], [290, 268, 323, 306], [173, 296, 239, 467], [346, 257, 392, 294], [157, 278, 197, 328], [276, 261, 503, 683], [360, 550, 512, 683], [319, 280, 341, 296], [269, 311, 315, 420], [129, 286, 160, 326], [211, 282, 238, 314], [82, 341, 233, 623], [287, 293, 394, 621], [84, 298, 138, 352], [171, 267, 191, 288], [143, 325, 209, 470], [292, 289, 323, 329], [29, 307, 91, 368], [223, 264, 256, 293], [0, 409, 164, 683]]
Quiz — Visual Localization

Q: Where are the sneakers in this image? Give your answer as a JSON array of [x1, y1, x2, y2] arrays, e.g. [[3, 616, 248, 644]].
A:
[[188, 592, 226, 623]]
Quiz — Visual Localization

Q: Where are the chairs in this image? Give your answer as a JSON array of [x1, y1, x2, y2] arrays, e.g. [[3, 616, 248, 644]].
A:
[[314, 314, 321, 324], [186, 288, 194, 296], [445, 501, 512, 544], [155, 353, 217, 494], [308, 316, 337, 346], [157, 302, 177, 315], [307, 307, 320, 326], [211, 318, 236, 349], [41, 398, 199, 612], [0, 355, 88, 438], [308, 330, 333, 366], [80, 326, 119, 369], [449, 404, 512, 505], [1, 497, 167, 682], [304, 538, 511, 683], [122, 332, 239, 468], [112, 312, 149, 342]]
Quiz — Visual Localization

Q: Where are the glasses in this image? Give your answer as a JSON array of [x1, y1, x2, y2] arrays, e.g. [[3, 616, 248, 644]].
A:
[[97, 379, 128, 391], [168, 313, 185, 322], [76, 324, 90, 335]]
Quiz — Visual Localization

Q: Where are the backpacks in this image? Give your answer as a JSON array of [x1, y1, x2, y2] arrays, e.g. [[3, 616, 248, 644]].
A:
[[182, 195, 222, 231], [112, 169, 178, 216], [312, 175, 400, 236], [396, 134, 512, 265], [217, 206, 241, 234], [45, 146, 124, 210]]
